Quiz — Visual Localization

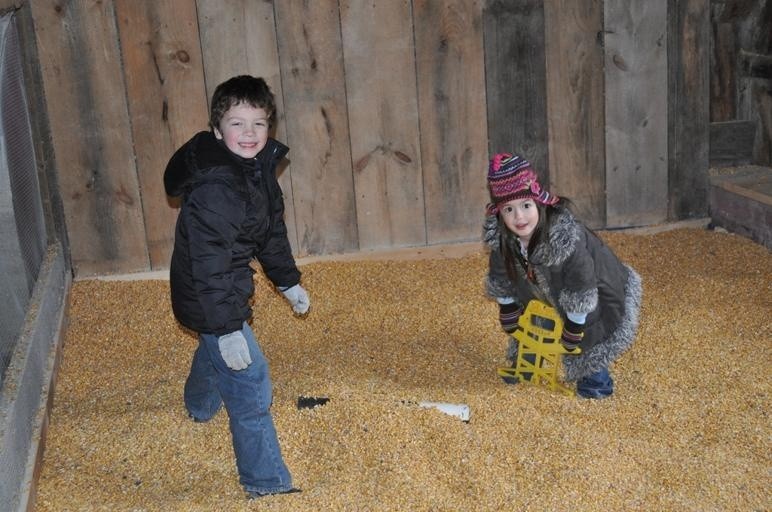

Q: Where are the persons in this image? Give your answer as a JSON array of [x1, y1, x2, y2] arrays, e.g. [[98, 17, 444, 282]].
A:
[[163, 74, 310, 499], [482, 152, 644, 398]]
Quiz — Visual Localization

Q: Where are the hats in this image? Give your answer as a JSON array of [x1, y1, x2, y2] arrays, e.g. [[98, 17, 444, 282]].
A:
[[487, 152, 540, 206]]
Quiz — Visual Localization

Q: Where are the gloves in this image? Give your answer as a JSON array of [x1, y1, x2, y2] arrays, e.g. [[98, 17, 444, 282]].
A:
[[217, 328, 253, 370], [561, 313, 587, 352], [497, 301, 521, 334], [276, 282, 311, 316]]
